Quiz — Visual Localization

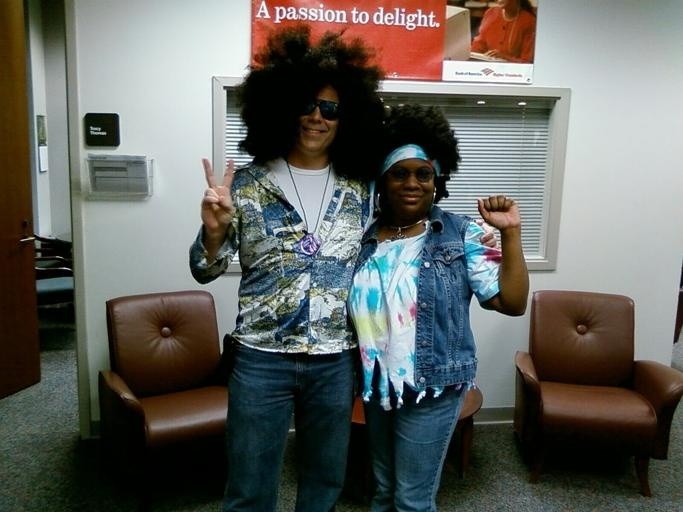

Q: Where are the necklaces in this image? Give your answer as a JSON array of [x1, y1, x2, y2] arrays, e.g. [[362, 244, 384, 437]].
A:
[[378, 219, 424, 243], [502, 8, 519, 21], [281, 156, 332, 236]]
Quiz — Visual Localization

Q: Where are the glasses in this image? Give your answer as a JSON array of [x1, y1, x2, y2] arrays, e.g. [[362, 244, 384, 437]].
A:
[[298, 97, 342, 122], [387, 164, 435, 183]]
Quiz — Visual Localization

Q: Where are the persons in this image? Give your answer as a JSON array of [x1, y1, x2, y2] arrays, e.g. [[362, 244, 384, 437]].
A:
[[183, 22, 497, 511], [346, 100, 532, 512], [469, 0, 536, 66]]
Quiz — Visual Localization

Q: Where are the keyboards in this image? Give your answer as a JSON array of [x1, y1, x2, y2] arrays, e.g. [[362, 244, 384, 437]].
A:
[[470, 52, 507, 62]]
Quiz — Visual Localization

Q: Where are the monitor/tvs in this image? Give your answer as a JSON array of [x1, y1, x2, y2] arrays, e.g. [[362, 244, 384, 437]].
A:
[[444, 5, 472, 61]]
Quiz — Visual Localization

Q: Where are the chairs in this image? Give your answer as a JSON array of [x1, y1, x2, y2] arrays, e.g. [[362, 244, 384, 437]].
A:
[[34, 235, 75, 332], [96, 291, 229, 512], [514, 290, 683, 496]]
